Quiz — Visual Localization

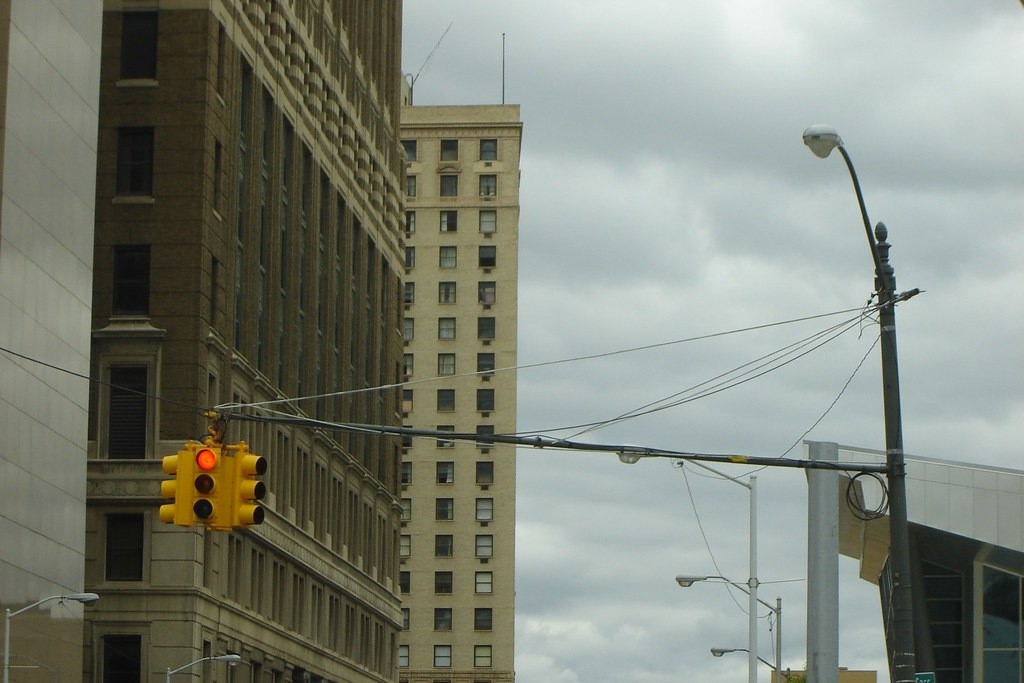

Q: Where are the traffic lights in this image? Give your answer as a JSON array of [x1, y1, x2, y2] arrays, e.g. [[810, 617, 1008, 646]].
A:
[[190, 445, 221, 526], [231, 449, 265, 531], [158, 450, 186, 528]]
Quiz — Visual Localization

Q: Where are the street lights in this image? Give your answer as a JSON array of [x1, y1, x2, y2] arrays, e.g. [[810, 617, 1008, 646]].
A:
[[710, 646, 792, 683], [614, 449, 759, 683], [800, 122, 924, 683], [675, 574, 783, 683]]
